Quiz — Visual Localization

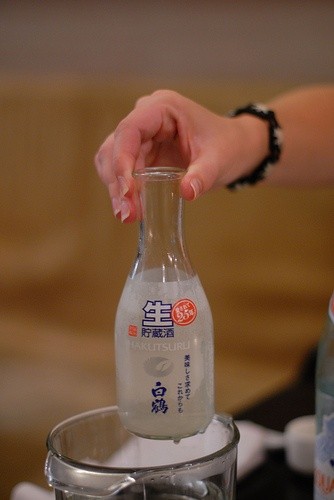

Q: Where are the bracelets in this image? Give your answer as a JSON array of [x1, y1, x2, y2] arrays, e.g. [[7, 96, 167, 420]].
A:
[[218, 101, 287, 192]]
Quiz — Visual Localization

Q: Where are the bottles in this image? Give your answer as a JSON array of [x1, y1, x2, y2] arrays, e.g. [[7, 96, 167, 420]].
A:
[[313, 290, 334, 500], [116, 167, 213, 441]]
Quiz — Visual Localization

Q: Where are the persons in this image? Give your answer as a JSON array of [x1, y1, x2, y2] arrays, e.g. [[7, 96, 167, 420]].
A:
[[92, 81, 334, 226]]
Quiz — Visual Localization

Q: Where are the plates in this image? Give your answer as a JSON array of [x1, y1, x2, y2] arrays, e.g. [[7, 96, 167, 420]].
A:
[[288, 419, 314, 469]]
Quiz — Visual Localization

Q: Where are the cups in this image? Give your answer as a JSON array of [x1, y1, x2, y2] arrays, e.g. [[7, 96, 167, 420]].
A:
[[45, 405, 241, 500]]
